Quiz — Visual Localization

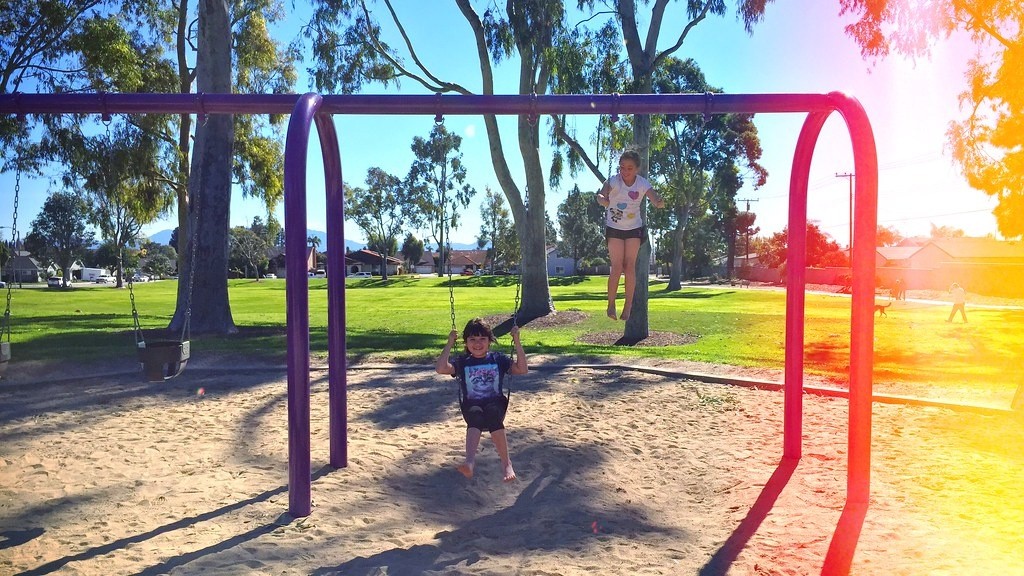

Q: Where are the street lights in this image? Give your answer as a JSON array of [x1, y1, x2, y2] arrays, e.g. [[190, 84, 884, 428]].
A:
[[0, 225, 22, 289]]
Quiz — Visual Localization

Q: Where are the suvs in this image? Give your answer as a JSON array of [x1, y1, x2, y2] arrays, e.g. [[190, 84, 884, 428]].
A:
[[348, 271, 372, 279], [47, 276, 73, 288]]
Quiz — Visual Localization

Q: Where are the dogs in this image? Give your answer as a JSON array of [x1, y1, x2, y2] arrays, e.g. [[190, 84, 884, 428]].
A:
[[874, 302, 892, 318]]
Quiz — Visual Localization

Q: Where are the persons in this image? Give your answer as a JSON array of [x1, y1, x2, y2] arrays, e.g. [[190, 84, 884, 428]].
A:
[[435, 316, 528, 483], [894, 278, 906, 300], [597, 150, 664, 321], [944, 283, 968, 323]]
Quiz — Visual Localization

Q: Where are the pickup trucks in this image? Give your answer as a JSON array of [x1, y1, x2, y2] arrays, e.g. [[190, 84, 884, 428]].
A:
[[95, 274, 117, 283]]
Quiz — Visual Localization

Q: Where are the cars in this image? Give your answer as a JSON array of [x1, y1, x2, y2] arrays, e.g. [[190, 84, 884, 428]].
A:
[[124, 271, 160, 282], [315, 268, 327, 278], [266, 274, 277, 279]]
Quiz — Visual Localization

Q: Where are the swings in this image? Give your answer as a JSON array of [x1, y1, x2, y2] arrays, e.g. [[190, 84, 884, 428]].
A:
[[605, 112, 712, 247], [100, 112, 209, 383], [0, 111, 23, 374], [435, 110, 538, 426]]
